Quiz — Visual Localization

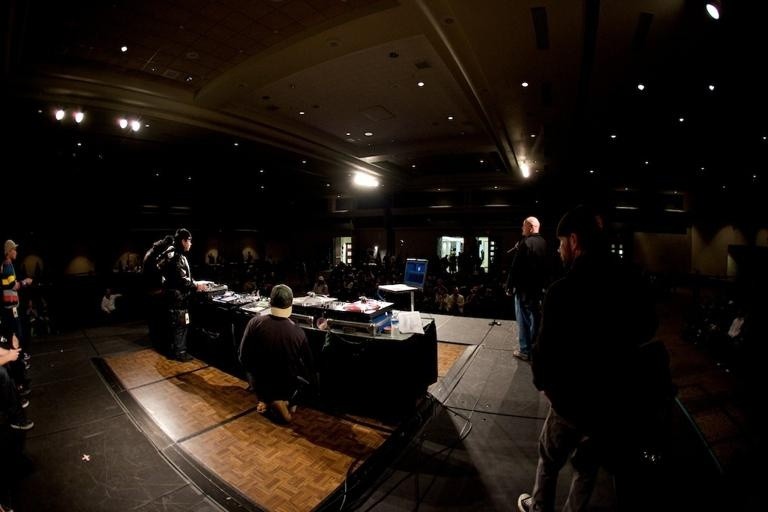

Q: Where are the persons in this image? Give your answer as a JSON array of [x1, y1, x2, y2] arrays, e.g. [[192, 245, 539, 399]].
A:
[[165, 227, 209, 361], [239, 284, 315, 423], [515, 200, 655, 512], [1, 237, 48, 431], [502, 215, 553, 361], [142, 235, 175, 353]]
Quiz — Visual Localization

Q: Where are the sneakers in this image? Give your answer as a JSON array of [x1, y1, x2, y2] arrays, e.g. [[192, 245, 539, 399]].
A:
[[23, 351, 30, 370], [274, 400, 291, 422], [9, 418, 35, 431], [517, 492, 535, 512], [514, 350, 529, 362], [20, 399, 30, 410], [257, 401, 268, 414]]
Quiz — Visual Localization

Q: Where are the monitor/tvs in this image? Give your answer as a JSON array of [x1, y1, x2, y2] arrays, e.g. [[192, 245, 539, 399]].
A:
[[404, 259, 429, 287]]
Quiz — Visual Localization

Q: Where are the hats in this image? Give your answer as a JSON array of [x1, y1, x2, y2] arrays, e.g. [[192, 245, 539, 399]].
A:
[[270, 284, 293, 317], [3, 239, 20, 255]]
